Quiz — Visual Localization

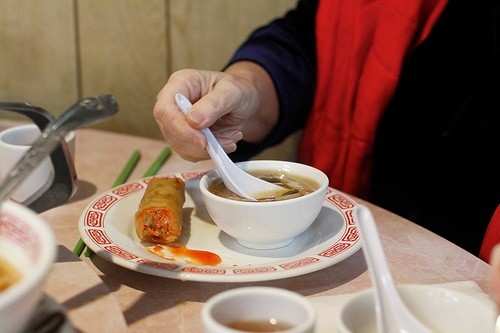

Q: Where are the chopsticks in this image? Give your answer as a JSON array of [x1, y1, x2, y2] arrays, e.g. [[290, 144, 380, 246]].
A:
[[73, 146, 172, 258]]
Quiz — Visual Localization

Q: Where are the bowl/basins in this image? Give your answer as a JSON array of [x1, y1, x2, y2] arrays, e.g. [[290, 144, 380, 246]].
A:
[[0, 199, 58, 332], [199, 159, 329, 251], [338, 282, 497, 333]]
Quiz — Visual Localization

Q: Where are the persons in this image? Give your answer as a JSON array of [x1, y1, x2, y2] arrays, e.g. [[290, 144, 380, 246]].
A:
[[153, 0, 500, 317]]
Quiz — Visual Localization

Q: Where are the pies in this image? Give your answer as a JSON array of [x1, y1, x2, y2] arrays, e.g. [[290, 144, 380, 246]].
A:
[[134, 177, 186, 244]]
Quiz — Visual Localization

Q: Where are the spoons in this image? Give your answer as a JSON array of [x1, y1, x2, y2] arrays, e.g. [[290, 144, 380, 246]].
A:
[[351, 202, 432, 333], [175, 92, 288, 200]]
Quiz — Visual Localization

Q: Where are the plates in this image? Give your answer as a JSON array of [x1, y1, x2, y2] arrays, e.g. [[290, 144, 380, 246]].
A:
[[77, 169, 365, 284]]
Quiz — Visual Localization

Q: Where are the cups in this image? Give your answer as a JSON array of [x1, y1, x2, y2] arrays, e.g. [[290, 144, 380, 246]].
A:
[[200, 286, 316, 333], [0, 124, 78, 202]]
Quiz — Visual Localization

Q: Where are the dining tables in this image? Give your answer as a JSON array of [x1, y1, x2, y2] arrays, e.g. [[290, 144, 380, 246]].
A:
[[0, 119, 500, 333]]
